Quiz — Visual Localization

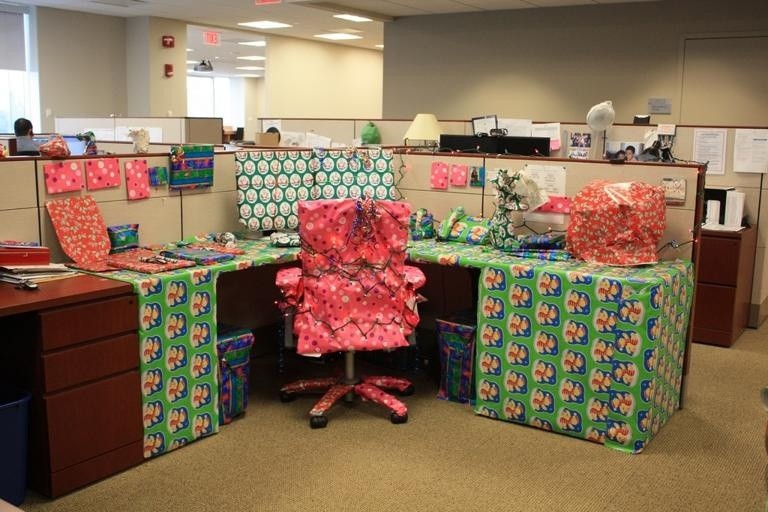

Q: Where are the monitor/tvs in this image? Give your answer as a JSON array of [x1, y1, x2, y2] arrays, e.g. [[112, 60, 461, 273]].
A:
[[440, 134, 549, 158], [51, 136, 87, 155], [32, 134, 51, 147]]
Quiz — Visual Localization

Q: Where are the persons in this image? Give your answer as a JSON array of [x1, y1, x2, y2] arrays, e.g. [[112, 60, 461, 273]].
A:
[[15, 118, 33, 137], [615, 146, 638, 161]]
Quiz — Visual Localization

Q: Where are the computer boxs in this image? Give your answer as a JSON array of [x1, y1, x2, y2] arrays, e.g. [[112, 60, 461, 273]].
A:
[[269, 298, 306, 398]]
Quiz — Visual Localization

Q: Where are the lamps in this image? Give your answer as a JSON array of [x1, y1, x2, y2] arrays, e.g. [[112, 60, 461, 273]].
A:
[[403, 112, 446, 150]]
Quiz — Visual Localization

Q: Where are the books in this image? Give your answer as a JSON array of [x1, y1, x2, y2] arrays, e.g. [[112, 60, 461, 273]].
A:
[[0, 262, 76, 284]]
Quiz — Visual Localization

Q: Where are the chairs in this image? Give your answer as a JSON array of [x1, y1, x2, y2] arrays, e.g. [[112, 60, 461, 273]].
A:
[[277, 198, 430, 429]]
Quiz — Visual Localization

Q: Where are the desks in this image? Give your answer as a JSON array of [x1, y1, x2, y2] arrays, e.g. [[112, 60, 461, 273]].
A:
[[0, 266, 144, 501], [66, 233, 695, 459]]
